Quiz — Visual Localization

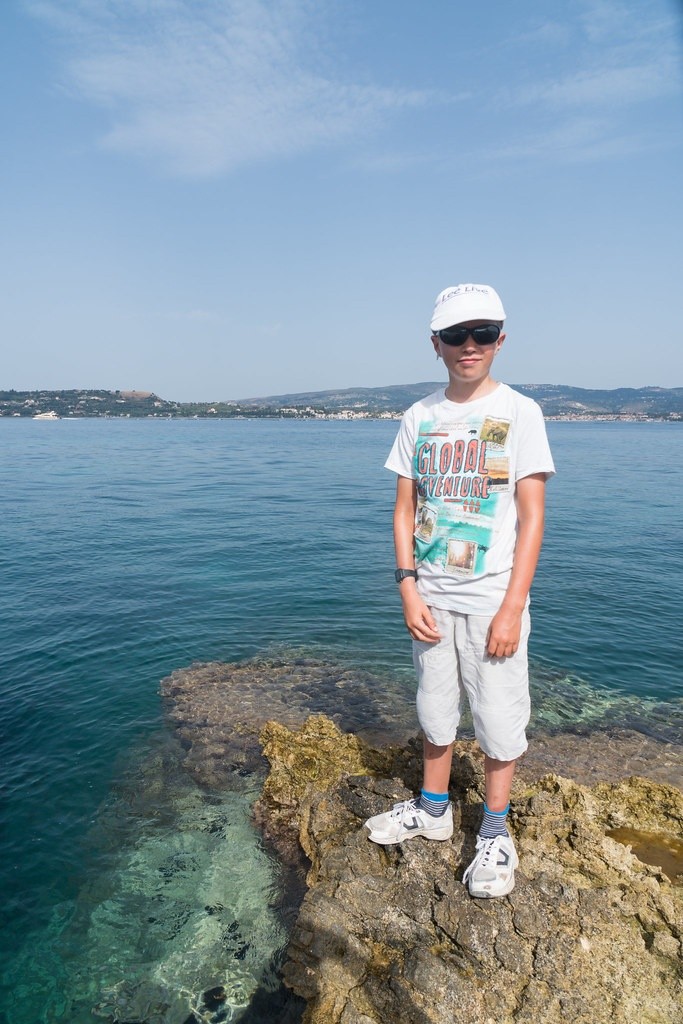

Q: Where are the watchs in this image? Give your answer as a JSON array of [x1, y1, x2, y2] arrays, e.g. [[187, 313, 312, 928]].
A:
[[394, 568, 417, 582]]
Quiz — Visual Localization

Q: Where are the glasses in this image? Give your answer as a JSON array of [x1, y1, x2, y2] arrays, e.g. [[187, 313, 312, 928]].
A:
[[431, 324, 506, 346]]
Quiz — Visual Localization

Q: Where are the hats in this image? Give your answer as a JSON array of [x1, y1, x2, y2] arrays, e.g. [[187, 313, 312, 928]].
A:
[[426, 283, 508, 331]]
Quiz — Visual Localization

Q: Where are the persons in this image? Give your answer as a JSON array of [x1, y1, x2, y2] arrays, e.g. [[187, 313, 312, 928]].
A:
[[358, 283, 554, 903]]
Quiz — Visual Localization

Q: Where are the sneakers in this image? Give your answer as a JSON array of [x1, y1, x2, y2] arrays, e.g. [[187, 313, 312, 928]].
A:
[[457, 832, 523, 900], [364, 797, 457, 847]]
